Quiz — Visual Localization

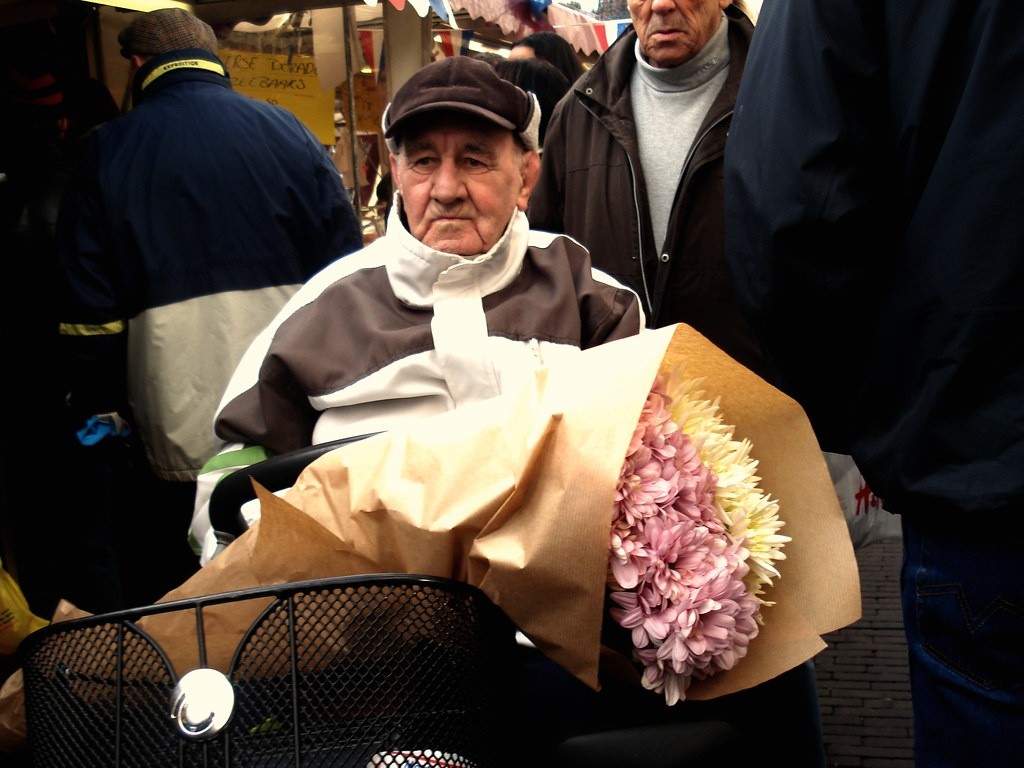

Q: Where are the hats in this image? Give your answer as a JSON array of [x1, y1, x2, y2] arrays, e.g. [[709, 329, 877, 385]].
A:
[[118, 7, 218, 58], [381, 55, 541, 152]]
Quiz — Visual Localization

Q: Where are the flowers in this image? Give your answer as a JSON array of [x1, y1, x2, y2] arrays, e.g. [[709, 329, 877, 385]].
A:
[[51, 323, 863, 708]]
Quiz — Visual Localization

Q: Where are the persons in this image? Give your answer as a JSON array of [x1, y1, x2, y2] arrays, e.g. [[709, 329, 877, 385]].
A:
[[53, 8, 364, 608], [185, 51, 648, 576], [525, 0, 759, 331], [714, 0, 1023, 767], [474, 31, 596, 165]]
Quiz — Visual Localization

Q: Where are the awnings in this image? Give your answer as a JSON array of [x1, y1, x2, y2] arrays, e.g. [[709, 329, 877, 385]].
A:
[[74, 0, 635, 81]]
